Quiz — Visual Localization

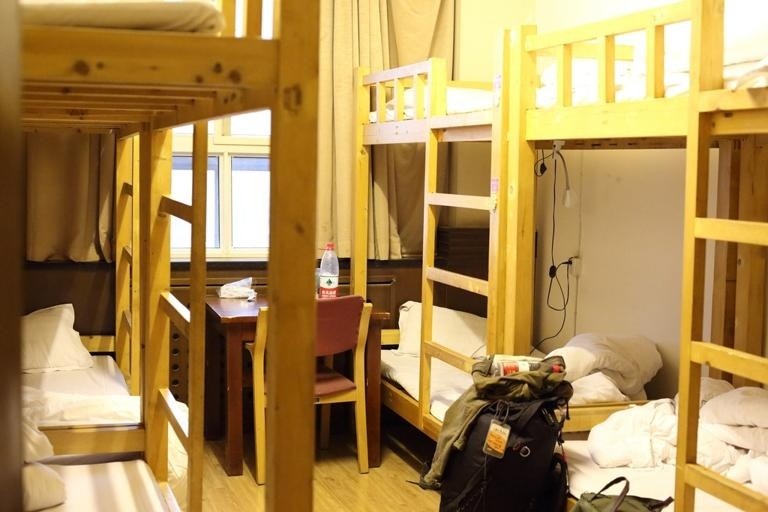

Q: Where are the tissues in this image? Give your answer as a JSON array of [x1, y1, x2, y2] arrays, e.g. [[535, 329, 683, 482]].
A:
[[217, 273, 256, 300]]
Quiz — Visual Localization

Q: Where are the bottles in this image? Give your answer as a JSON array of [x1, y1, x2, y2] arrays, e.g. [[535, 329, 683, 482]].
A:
[[490, 359, 560, 377], [319, 242, 340, 298]]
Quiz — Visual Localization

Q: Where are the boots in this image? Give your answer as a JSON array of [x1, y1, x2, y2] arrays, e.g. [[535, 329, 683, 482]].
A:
[[419, 461, 442, 489]]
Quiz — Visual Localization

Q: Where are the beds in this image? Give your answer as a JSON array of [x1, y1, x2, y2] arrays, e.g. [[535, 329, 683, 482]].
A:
[[2, 0, 322, 511], [351, 31, 508, 471], [509, 2, 767, 505]]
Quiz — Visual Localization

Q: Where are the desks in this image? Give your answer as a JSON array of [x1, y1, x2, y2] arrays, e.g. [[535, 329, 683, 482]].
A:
[[202, 296, 389, 476]]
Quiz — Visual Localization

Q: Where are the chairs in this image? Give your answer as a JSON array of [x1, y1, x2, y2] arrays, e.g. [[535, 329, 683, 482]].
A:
[[245, 294, 367, 483]]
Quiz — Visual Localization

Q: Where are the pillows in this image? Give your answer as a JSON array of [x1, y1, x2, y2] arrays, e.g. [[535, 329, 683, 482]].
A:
[[397, 300, 488, 356], [21, 302, 90, 372], [588, 398, 674, 466]]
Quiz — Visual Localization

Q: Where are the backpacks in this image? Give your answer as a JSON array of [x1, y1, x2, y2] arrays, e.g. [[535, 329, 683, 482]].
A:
[[439, 381, 574, 512]]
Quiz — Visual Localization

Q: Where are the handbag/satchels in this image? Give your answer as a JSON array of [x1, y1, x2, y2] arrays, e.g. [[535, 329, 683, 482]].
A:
[[567, 478, 674, 512]]
